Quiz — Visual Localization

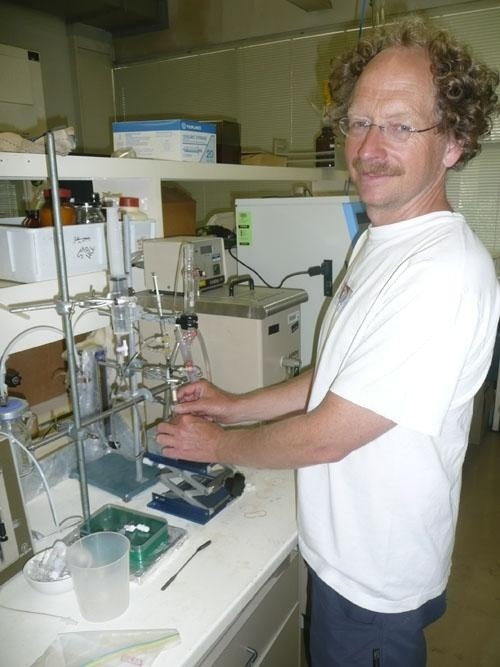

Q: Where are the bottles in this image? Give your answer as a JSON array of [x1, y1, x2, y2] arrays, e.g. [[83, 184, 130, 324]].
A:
[[21, 188, 148, 228], [315, 126, 338, 167], [182, 243, 196, 316]]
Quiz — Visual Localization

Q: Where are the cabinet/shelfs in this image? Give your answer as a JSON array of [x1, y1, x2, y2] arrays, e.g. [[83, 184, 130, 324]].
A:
[[0, 154, 352, 667]]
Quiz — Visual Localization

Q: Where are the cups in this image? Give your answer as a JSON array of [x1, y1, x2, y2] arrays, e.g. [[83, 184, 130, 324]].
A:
[[64, 531, 131, 623]]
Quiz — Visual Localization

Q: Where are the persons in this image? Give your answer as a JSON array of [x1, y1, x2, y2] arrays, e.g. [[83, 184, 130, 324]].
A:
[[147, 9, 500, 666]]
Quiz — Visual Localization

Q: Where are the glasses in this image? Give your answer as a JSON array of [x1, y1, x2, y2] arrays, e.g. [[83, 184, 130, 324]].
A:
[[339, 117, 442, 143]]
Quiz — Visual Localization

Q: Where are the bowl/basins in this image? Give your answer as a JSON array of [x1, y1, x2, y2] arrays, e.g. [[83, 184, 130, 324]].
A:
[[22, 546, 93, 595]]
